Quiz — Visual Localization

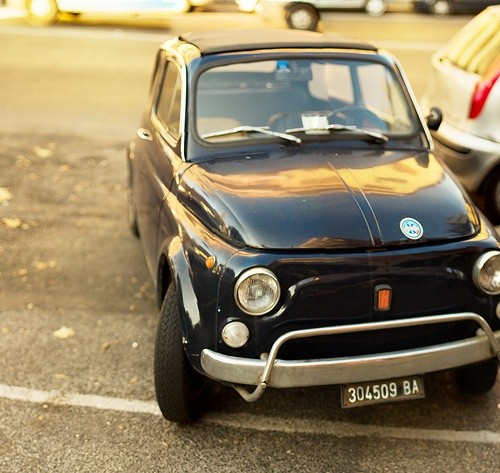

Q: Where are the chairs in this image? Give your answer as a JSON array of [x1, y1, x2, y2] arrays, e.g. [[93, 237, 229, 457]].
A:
[[268, 87, 333, 133], [171, 116, 246, 141]]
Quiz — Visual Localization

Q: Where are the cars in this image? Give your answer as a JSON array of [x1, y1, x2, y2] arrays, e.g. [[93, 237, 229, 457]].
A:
[[127, 30, 500, 423], [418, 5, 500, 225], [0, 1, 500, 31]]
